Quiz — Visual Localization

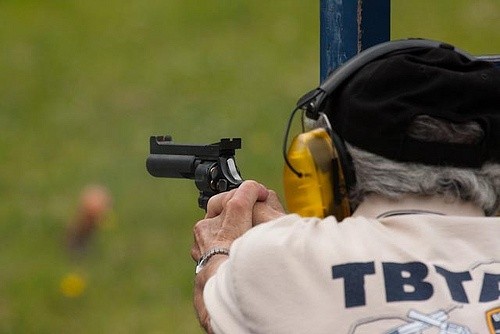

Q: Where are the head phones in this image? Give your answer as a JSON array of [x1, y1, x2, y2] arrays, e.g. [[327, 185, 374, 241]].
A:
[[283, 38, 479, 223]]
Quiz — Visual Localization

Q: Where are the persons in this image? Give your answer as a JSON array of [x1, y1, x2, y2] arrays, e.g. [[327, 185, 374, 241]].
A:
[[189, 36, 500, 334]]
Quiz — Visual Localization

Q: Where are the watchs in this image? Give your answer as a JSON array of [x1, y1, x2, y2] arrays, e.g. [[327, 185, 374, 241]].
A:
[[194, 246, 230, 274]]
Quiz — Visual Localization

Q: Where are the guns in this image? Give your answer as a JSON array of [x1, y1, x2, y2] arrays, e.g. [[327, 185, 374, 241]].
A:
[[146, 134, 246, 213]]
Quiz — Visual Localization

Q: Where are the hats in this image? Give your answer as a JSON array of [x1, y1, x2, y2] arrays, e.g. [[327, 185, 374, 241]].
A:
[[318, 37, 500, 169]]
